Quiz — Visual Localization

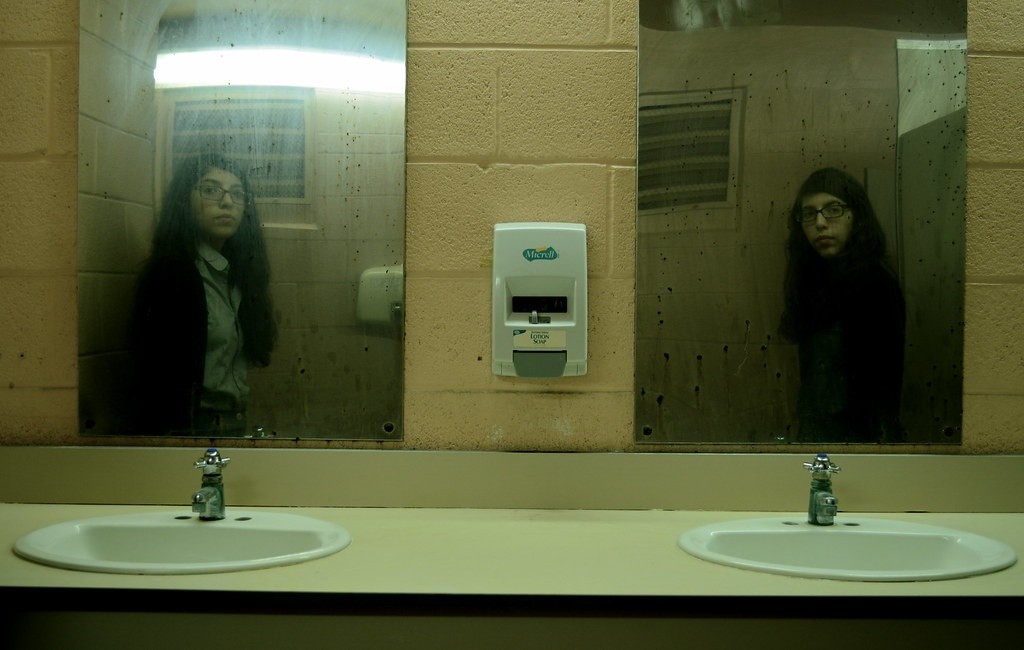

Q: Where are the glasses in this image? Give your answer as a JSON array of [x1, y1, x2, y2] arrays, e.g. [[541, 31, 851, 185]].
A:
[[190, 184, 253, 206], [794, 204, 848, 224]]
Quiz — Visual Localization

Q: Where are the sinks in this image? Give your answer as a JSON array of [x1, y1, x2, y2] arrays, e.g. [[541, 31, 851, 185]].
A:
[[12, 510, 350, 574], [677, 517, 1017, 582]]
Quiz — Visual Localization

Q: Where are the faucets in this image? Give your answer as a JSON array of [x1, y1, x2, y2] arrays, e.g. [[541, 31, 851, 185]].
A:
[[772, 435, 787, 443], [192, 449, 230, 520], [803, 453, 841, 526], [244, 425, 272, 438]]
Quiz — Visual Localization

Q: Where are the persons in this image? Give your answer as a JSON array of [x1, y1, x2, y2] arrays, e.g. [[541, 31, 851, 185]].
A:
[[120, 154, 277, 436], [776, 167, 905, 444]]
